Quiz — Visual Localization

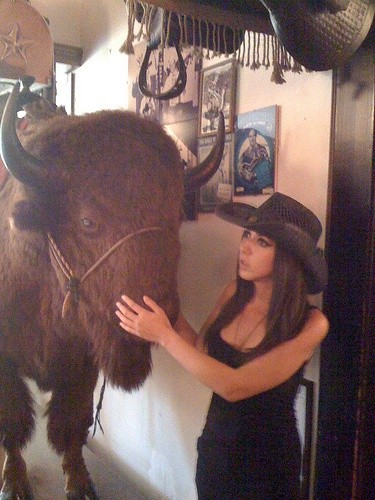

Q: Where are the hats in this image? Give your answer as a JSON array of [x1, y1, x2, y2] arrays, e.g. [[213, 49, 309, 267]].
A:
[[216, 192, 329, 294]]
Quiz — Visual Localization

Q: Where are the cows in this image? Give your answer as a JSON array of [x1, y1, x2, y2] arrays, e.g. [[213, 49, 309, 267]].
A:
[[0, 81, 226, 500]]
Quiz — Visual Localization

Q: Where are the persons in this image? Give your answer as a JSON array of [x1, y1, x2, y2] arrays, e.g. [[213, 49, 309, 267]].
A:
[[207, 80, 228, 131], [237, 128, 274, 190], [139, 58, 186, 120], [115, 191, 330, 500]]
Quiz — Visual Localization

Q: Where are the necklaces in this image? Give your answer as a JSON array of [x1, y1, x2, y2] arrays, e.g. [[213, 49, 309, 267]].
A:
[[233, 308, 267, 353]]
